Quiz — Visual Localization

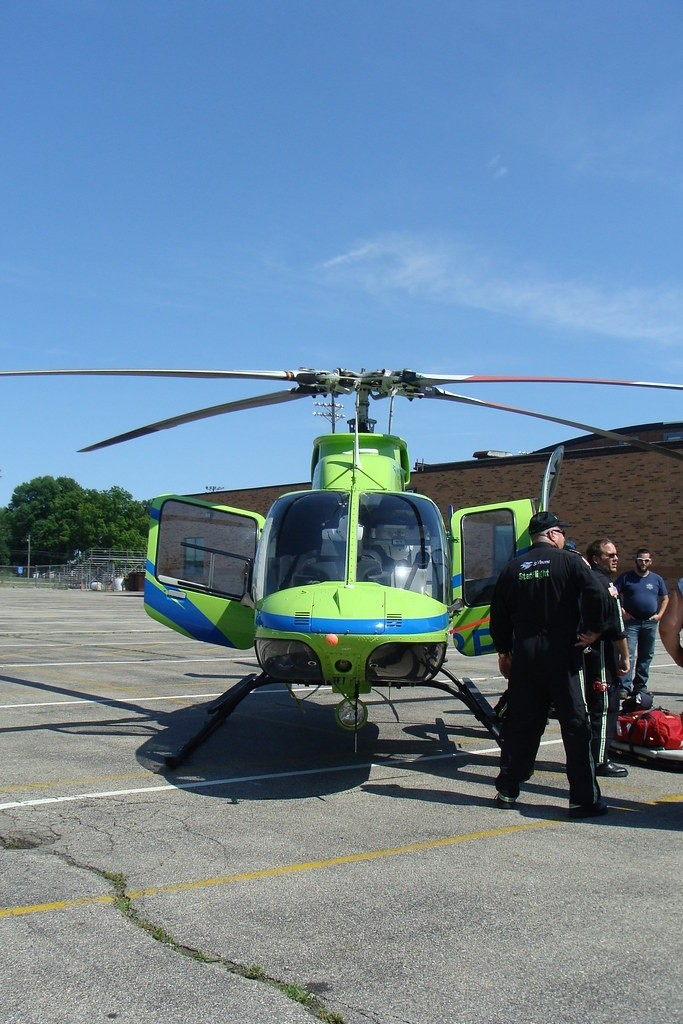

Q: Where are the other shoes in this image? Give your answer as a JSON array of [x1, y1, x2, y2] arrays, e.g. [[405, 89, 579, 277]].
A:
[[568, 801, 608, 819], [497, 792, 517, 809], [620, 689, 628, 699]]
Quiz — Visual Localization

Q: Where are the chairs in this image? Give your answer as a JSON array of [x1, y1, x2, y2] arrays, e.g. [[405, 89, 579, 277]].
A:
[[290, 528, 381, 586]]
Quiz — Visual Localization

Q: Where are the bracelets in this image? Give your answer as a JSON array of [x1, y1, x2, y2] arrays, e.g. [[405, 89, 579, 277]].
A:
[[499, 653, 508, 657]]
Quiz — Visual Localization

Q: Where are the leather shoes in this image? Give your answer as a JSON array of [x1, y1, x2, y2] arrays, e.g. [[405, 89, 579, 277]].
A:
[[596, 759, 628, 777]]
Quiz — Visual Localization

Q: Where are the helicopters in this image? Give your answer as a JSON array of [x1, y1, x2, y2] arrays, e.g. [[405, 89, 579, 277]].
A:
[[0, 365, 683, 771]]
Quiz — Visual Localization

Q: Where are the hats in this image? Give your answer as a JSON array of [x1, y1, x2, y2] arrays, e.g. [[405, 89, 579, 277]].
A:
[[529, 511, 572, 535]]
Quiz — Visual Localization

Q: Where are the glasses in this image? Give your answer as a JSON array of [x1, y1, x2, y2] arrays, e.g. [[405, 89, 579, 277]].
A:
[[546, 529, 566, 538], [636, 558, 651, 563], [609, 554, 618, 558]]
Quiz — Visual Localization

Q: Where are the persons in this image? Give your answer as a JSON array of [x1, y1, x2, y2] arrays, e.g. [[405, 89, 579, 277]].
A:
[[490, 511, 608, 819], [562, 539, 683, 777]]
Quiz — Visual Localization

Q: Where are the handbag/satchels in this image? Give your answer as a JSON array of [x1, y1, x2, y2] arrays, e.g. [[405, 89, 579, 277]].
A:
[[613, 706, 683, 749], [622, 691, 654, 710]]
